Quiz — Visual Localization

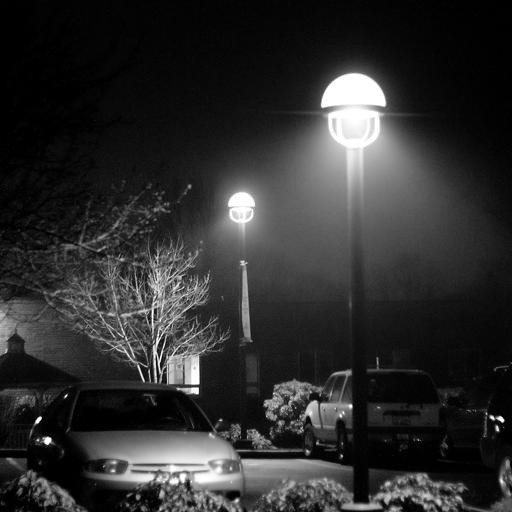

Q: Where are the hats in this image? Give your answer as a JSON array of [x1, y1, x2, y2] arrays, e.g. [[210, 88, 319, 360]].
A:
[[27, 381, 245, 504], [445, 365, 512, 498]]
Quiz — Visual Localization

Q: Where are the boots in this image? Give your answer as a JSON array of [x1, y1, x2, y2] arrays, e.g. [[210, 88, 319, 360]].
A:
[[302, 369, 442, 464]]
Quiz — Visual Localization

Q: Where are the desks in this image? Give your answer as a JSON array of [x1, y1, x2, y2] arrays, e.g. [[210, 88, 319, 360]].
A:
[[228, 191, 256, 450], [321, 74, 387, 504]]
